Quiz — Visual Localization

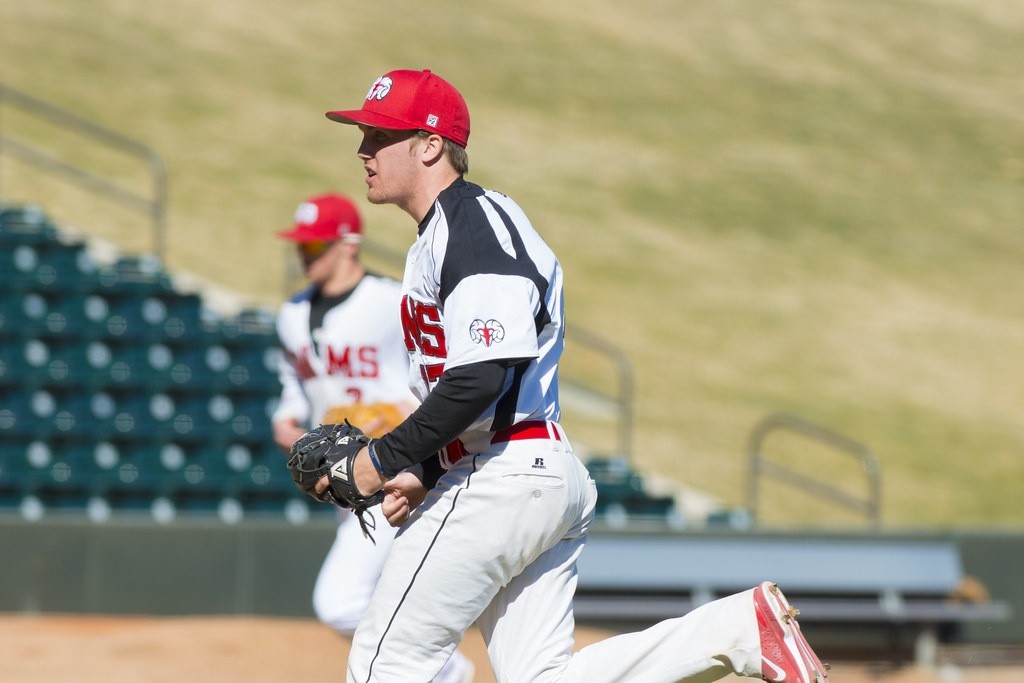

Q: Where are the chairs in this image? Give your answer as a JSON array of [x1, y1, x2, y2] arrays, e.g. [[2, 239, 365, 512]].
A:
[[0, 207, 336, 516]]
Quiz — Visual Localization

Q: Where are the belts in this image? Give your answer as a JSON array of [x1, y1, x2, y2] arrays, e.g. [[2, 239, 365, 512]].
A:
[[440, 421, 561, 466]]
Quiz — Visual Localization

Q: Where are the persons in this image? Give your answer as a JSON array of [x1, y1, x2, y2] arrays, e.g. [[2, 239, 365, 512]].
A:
[[314, 66, 830, 683], [269, 194, 474, 683]]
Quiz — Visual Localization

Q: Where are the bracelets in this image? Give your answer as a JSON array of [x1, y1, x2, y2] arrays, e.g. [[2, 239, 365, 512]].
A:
[[368, 442, 384, 475]]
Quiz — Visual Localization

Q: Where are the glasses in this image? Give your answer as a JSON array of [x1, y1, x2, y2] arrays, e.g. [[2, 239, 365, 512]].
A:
[[296, 240, 341, 264]]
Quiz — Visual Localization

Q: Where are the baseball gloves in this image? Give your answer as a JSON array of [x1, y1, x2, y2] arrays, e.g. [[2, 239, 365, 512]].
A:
[[288, 422, 376, 513]]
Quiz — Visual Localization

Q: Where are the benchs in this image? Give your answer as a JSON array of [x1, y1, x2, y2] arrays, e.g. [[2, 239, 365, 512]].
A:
[[575, 538, 1010, 666]]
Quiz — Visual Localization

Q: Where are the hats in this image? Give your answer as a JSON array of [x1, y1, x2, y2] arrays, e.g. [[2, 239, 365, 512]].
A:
[[326, 68, 471, 148], [277, 195, 361, 241]]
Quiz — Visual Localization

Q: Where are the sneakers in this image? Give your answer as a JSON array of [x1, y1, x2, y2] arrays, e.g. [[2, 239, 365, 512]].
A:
[[752, 581, 830, 683]]
